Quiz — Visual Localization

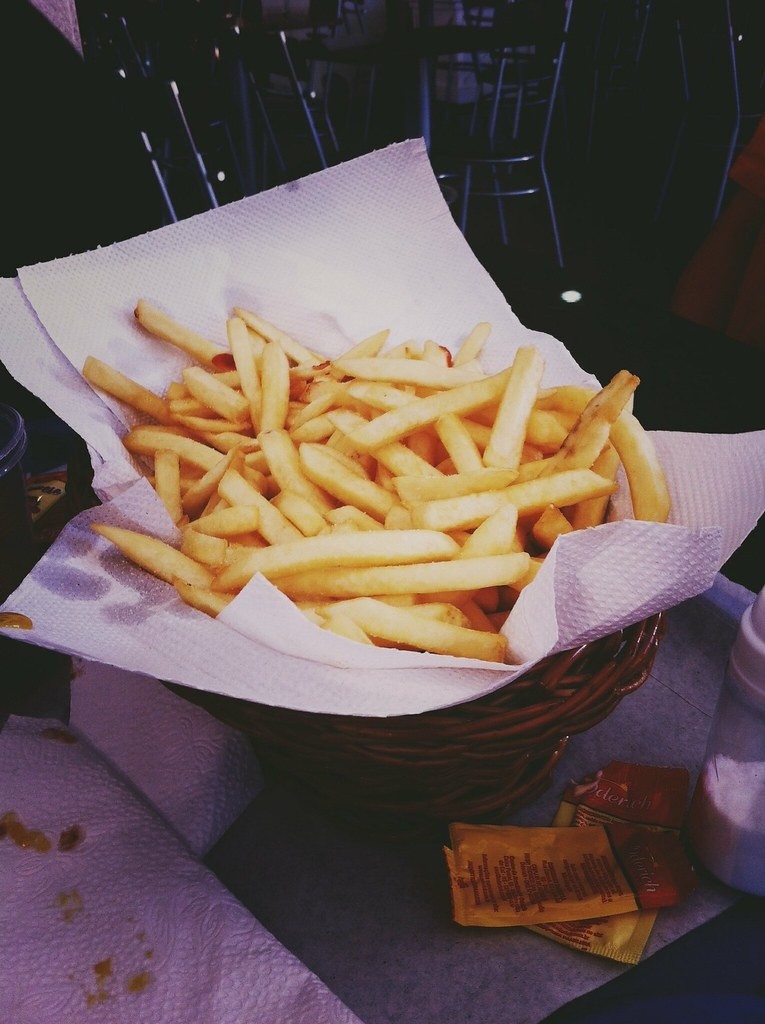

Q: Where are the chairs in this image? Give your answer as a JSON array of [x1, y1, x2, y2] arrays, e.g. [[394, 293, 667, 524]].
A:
[[97, 0, 765, 272]]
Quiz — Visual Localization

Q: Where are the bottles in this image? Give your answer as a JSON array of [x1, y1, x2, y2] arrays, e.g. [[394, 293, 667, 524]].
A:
[[689, 584, 765, 897]]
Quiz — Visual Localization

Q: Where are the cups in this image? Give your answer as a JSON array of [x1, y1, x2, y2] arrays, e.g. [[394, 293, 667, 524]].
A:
[[0, 401, 33, 699]]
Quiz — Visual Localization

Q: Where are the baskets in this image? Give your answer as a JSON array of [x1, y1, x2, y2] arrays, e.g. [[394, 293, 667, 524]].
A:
[[62, 470, 666, 824]]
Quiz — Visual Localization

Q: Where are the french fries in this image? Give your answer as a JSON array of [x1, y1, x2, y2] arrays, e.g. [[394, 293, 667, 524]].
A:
[[83, 300, 670, 664]]
